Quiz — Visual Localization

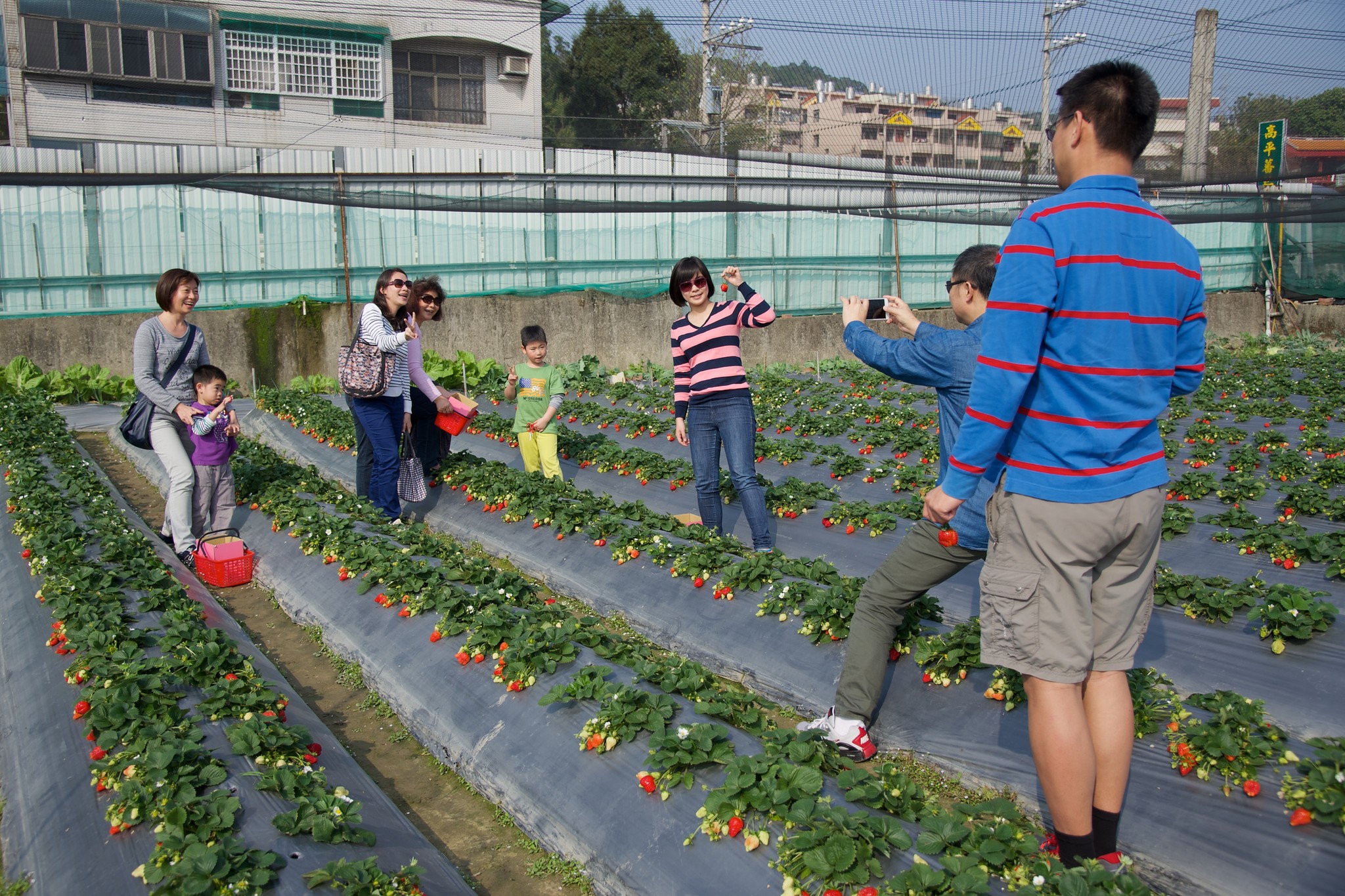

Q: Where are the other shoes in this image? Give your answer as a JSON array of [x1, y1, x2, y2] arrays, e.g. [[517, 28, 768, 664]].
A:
[[430, 467, 442, 475], [392, 519, 406, 531], [755, 548, 773, 554]]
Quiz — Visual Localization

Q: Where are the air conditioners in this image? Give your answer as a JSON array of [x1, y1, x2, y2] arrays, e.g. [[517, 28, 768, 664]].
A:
[[500, 56, 529, 76]]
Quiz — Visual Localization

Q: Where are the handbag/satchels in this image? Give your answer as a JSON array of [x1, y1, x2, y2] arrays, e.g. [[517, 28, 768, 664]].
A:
[[118, 391, 155, 449], [338, 304, 396, 398], [397, 432, 427, 503]]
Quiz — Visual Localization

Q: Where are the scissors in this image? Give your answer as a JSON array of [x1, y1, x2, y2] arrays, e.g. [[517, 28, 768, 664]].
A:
[[526, 423, 537, 440]]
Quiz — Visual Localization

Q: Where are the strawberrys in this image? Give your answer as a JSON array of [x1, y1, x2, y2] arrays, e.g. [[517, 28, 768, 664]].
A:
[[721, 276, 728, 292], [0, 360, 1345, 896]]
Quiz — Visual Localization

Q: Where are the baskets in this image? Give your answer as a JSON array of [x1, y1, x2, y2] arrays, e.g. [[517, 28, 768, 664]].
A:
[[434, 408, 479, 436], [192, 527, 254, 587]]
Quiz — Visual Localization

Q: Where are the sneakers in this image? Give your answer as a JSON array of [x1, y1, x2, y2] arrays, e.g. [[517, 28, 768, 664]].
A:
[[796, 706, 878, 762], [177, 544, 197, 568], [159, 533, 175, 545], [1013, 833, 1127, 883]]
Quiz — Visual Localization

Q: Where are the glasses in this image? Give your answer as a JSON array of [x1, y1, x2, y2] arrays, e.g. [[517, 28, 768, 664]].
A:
[[945, 279, 977, 295], [679, 277, 707, 292], [386, 279, 413, 290], [419, 294, 442, 306], [1045, 113, 1091, 142]]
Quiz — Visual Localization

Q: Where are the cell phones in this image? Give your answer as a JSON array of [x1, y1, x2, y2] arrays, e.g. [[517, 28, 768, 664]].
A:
[[860, 298, 889, 321]]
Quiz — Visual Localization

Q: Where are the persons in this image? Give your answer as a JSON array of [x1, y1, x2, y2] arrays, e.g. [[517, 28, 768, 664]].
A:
[[921, 56, 1208, 868], [345, 268, 455, 533], [185, 365, 239, 546], [788, 245, 1011, 765], [133, 268, 211, 570], [669, 257, 777, 555], [504, 325, 565, 484]]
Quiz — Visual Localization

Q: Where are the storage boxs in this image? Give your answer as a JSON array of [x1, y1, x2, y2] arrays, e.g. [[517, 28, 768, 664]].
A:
[[196, 534, 245, 578], [447, 392, 479, 417]]
